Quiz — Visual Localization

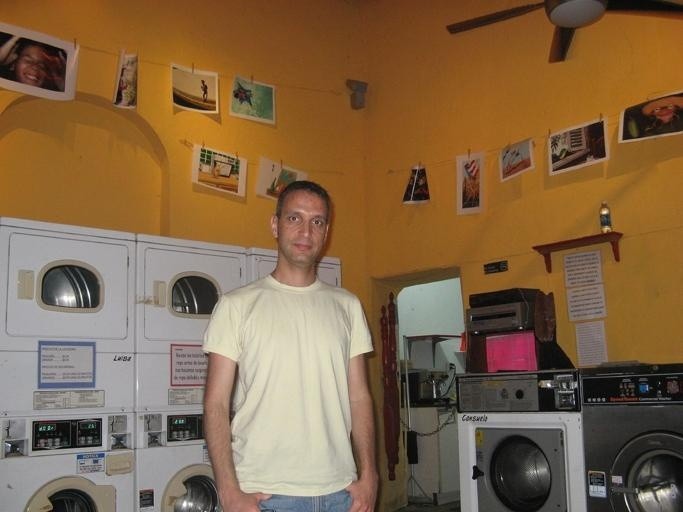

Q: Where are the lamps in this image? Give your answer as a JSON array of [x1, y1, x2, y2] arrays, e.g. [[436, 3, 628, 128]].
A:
[[546, 0, 607, 28]]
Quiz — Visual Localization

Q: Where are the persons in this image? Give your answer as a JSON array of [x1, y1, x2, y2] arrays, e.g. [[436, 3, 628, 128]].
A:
[[0, 31, 66, 94], [114, 66, 130, 105], [200, 180, 380, 510], [199, 79, 210, 104]]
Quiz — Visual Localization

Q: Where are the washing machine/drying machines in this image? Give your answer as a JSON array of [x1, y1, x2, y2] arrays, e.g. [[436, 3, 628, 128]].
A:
[[455, 368, 586, 512], [583, 362, 683, 512], [1, 217, 344, 512]]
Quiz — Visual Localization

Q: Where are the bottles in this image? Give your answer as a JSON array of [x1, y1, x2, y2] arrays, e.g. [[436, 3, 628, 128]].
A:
[[599, 201, 612, 234]]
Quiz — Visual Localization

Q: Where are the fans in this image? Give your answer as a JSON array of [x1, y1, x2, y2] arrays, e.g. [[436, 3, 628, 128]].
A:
[[447, 0, 683, 63]]
